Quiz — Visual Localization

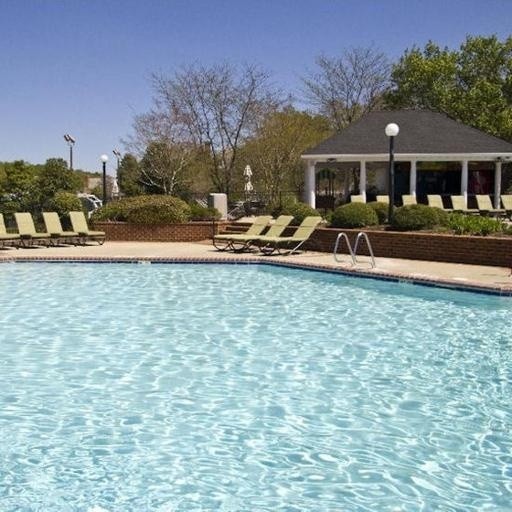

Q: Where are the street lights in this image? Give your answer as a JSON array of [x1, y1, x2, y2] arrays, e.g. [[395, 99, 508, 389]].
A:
[[100, 154, 108, 206], [63, 134, 75, 170], [384, 122, 401, 224]]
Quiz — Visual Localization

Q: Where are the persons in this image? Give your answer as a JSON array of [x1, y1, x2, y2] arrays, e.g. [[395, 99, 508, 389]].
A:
[[333, 193, 346, 208]]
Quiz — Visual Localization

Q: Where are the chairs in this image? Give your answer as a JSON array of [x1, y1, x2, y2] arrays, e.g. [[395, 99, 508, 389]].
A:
[[0, 211, 106, 249], [349, 194, 512, 222], [213, 215, 323, 256]]
